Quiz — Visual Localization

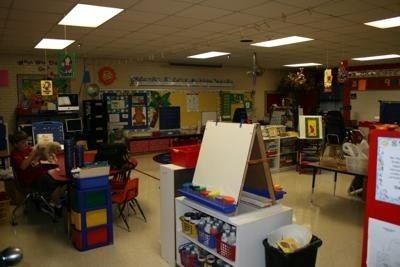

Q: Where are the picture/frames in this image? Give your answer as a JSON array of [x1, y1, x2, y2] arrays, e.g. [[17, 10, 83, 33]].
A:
[[17, 74, 49, 109]]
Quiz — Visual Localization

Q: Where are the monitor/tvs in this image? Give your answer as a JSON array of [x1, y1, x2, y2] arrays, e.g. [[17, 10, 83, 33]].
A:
[[159, 105, 181, 131], [57, 93, 80, 111]]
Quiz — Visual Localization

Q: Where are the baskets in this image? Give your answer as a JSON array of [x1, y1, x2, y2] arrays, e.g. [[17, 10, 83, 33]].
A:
[[179, 215, 198, 239], [216, 236, 235, 263], [168, 144, 202, 168], [196, 225, 216, 250]]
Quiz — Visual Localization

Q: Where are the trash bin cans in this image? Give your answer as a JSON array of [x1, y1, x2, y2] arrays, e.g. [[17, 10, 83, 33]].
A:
[[263, 235, 322, 267]]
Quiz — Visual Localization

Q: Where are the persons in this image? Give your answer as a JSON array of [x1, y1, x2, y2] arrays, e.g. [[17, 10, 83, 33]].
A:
[[9, 130, 64, 208]]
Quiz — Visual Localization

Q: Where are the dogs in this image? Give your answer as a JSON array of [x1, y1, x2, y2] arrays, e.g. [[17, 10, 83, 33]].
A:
[[31, 142, 61, 166]]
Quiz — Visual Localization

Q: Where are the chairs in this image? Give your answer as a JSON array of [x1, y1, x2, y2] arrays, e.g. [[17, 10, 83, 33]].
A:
[[9, 163, 60, 226], [111, 177, 147, 232], [323, 110, 353, 145]]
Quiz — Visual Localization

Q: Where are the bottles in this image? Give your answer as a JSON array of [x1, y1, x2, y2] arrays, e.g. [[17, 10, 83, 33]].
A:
[[179, 210, 236, 266]]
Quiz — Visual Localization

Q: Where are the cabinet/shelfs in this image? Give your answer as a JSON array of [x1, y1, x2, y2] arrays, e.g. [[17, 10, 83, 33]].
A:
[[264, 135, 299, 172], [175, 196, 293, 267]]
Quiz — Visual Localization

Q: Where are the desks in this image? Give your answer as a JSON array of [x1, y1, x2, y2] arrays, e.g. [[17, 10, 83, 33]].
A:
[[305, 161, 368, 203]]
